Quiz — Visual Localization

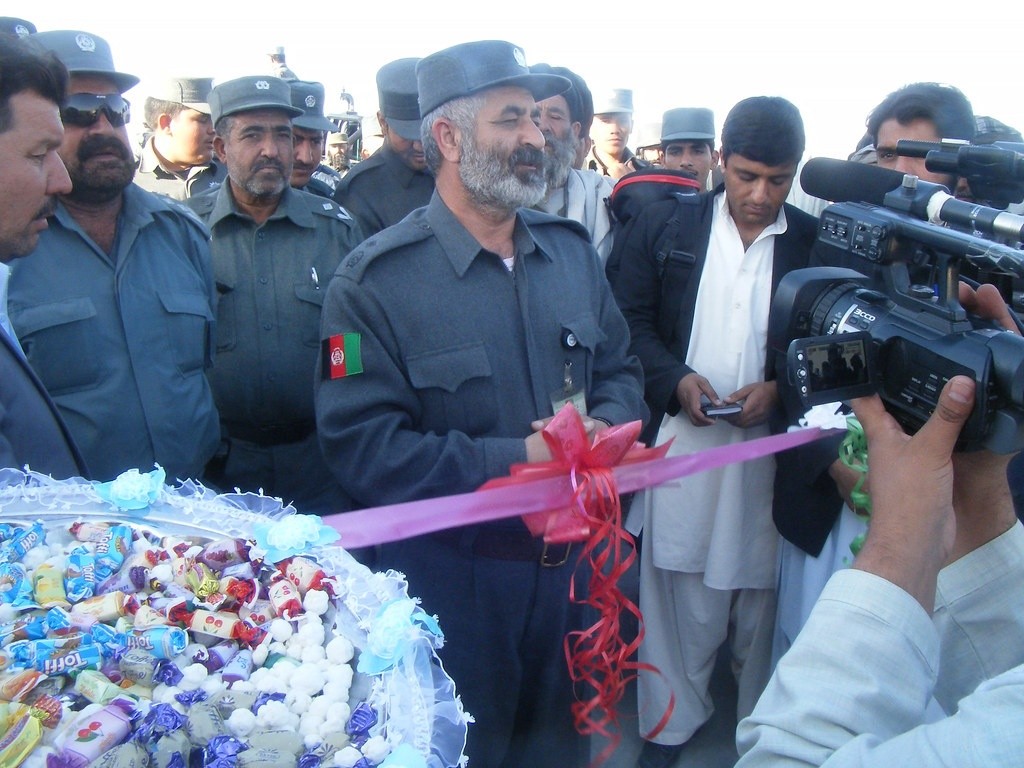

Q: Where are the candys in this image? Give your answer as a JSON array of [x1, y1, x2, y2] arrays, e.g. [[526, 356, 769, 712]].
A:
[[0, 519, 391, 768]]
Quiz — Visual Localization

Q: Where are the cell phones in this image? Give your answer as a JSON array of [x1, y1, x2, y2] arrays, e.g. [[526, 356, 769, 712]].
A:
[[701, 403, 743, 416]]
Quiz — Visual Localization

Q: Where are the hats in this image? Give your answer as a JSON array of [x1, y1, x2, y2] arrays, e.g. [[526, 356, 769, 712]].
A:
[[149, 75, 215, 114], [283, 78, 339, 132], [29, 30, 140, 94], [206, 76, 304, 130], [593, 89, 633, 115], [266, 47, 285, 56], [415, 40, 573, 119], [0, 16, 38, 38], [662, 107, 715, 140], [377, 58, 424, 140], [327, 132, 350, 145]]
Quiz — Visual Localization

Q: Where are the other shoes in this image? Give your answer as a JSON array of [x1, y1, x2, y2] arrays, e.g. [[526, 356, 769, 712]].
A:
[[638, 740, 685, 768]]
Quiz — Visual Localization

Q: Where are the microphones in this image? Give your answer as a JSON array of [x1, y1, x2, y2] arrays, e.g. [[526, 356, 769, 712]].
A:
[[800, 157, 953, 220]]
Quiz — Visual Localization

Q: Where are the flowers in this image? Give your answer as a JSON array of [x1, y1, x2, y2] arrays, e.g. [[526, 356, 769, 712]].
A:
[[474, 402, 678, 768]]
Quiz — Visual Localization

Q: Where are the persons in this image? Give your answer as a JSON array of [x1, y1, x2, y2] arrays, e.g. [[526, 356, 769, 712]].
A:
[[604, 95, 821, 767], [735, 282, 1024, 767], [807, 343, 870, 390], [313, 39, 645, 768], [1, 16, 1024, 768]]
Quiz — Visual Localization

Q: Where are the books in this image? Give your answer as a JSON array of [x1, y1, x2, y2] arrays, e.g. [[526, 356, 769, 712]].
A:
[[702, 401, 744, 415]]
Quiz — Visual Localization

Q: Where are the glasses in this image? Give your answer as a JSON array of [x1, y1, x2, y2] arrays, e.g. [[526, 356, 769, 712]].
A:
[[61, 93, 131, 127]]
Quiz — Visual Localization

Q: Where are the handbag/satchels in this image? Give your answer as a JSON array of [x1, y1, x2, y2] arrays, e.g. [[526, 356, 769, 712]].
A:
[[603, 168, 703, 283]]
